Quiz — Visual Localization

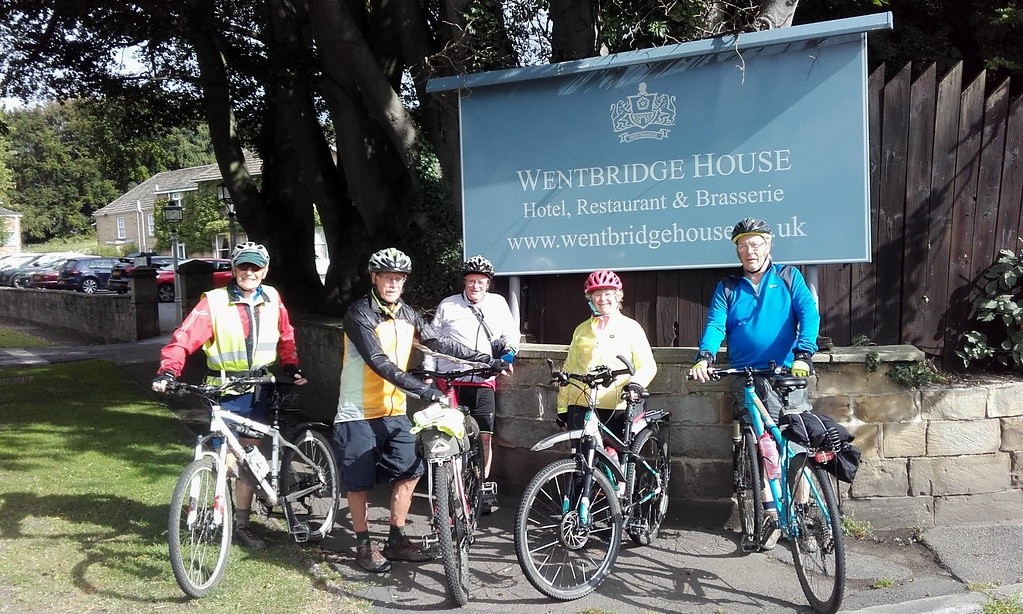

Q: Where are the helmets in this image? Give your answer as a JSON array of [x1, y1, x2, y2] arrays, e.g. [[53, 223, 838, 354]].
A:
[[584, 270, 622, 295], [732, 218, 772, 244], [461, 255, 495, 279], [368, 247, 412, 276], [230, 242, 270, 261]]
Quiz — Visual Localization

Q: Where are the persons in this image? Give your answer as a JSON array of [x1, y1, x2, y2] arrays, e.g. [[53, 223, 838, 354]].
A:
[[333, 248, 513, 571], [422, 255, 522, 515], [151, 241, 306, 550], [689, 217, 821, 552], [556, 271, 658, 539]]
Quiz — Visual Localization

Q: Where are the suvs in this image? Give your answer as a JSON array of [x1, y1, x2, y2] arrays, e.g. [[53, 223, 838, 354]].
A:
[[105, 251, 187, 296], [57, 257, 122, 294], [156, 257, 234, 303]]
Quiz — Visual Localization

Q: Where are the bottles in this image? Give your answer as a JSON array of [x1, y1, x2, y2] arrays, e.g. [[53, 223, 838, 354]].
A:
[[245, 445, 270, 475], [759, 431, 782, 479], [605, 446, 620, 466]]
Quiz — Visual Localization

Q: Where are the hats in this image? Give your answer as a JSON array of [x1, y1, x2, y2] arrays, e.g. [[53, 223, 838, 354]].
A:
[[234, 252, 267, 267]]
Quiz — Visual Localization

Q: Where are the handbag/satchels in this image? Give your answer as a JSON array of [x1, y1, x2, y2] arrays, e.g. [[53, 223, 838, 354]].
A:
[[414, 424, 470, 460], [491, 338, 506, 360]]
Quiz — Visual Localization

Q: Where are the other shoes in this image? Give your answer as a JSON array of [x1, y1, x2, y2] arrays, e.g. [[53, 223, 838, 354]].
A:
[[605, 508, 628, 541], [477, 500, 491, 515]]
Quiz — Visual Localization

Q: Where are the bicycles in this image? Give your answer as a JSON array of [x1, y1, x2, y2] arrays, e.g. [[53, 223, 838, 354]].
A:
[[685, 359, 847, 614], [160, 374, 342, 601], [408, 367, 499, 608], [513, 354, 672, 602]]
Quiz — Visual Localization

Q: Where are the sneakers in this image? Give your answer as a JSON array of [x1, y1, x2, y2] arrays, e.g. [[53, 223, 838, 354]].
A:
[[758, 510, 783, 549], [798, 513, 818, 552], [382, 536, 432, 562], [354, 539, 391, 573], [235, 523, 266, 550]]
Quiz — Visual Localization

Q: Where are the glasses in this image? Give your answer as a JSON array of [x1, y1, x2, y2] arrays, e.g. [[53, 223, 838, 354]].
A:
[[468, 279, 485, 286], [737, 242, 765, 252], [237, 263, 261, 272], [377, 272, 407, 284]]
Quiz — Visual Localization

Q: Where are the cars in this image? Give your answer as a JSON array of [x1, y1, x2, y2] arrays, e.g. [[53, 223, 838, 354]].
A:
[[0, 252, 88, 289]]
